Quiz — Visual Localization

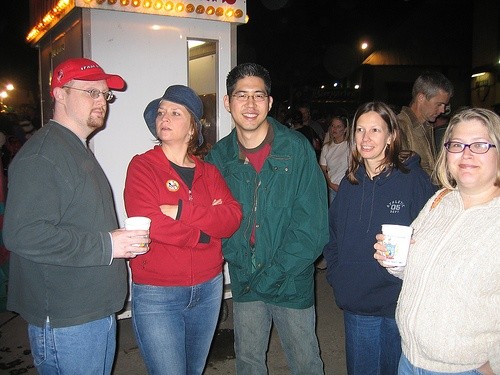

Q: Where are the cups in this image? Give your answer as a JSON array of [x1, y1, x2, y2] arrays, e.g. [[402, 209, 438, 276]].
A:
[[125, 217, 151, 254], [382, 224, 413, 266]]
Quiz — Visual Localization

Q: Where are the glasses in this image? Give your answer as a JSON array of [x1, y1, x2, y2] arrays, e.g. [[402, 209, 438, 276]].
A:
[[444, 141, 498, 154], [230, 92, 267, 102], [62, 85, 115, 101]]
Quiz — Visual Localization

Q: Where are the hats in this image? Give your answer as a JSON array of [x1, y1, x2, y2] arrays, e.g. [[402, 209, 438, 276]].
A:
[[48, 58, 126, 96], [144, 85, 206, 146]]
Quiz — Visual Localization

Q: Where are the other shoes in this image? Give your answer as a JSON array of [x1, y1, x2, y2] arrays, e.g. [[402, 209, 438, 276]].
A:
[[316, 257, 329, 270]]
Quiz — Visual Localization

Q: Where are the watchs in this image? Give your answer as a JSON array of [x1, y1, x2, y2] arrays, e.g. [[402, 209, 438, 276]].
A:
[[122, 84, 243, 375]]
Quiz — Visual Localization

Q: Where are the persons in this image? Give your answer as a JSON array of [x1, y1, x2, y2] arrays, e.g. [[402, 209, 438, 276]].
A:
[[0, 58, 152, 375], [205, 63, 500, 375]]
